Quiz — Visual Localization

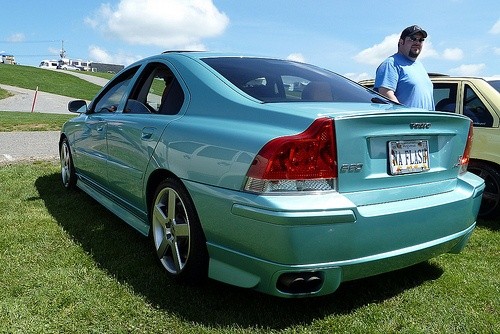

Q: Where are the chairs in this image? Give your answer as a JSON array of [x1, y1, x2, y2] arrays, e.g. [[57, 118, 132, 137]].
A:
[[301, 81, 333, 101], [435, 98, 456, 111]]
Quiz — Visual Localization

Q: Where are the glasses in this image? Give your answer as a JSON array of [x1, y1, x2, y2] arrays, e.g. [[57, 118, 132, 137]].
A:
[[405, 37, 424, 42]]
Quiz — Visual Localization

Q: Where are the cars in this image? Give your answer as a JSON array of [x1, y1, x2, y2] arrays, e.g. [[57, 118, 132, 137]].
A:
[[57, 64, 80, 71], [359, 74, 500, 224], [58, 51, 487, 301]]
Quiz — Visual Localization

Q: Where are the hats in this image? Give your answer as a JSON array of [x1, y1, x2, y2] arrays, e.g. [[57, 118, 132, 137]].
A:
[[400, 25, 427, 38]]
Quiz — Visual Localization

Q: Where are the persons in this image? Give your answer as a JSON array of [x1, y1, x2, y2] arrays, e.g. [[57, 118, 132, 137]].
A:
[[107, 105, 117, 112], [373, 25, 435, 111]]
